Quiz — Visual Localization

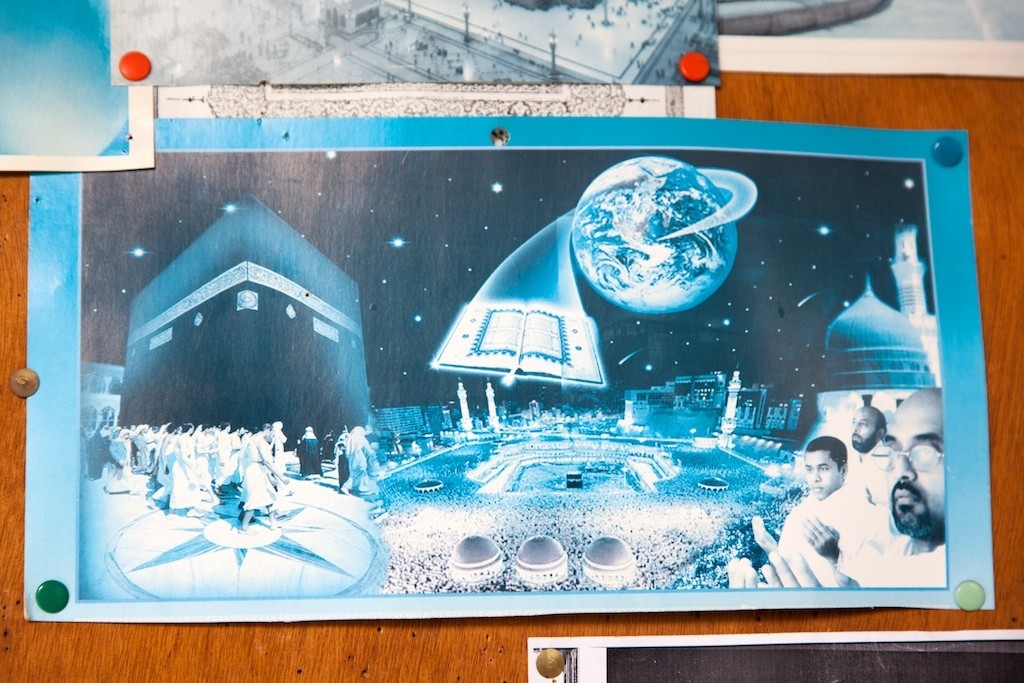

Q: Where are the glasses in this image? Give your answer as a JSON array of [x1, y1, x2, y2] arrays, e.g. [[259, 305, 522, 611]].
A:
[[871, 445, 945, 472]]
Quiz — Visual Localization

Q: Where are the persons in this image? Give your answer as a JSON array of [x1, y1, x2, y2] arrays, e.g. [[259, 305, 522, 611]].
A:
[[104, 428, 142, 495], [334, 432, 350, 494], [845, 405, 887, 503], [238, 421, 290, 535], [728, 386, 947, 590], [322, 428, 336, 463], [298, 426, 324, 478], [129, 424, 159, 474], [80, 421, 124, 481], [214, 421, 245, 495], [341, 426, 376, 496], [751, 435, 847, 588], [148, 422, 225, 518]]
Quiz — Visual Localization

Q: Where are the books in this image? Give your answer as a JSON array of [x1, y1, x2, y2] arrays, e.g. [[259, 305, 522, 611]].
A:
[[434, 299, 604, 383]]
